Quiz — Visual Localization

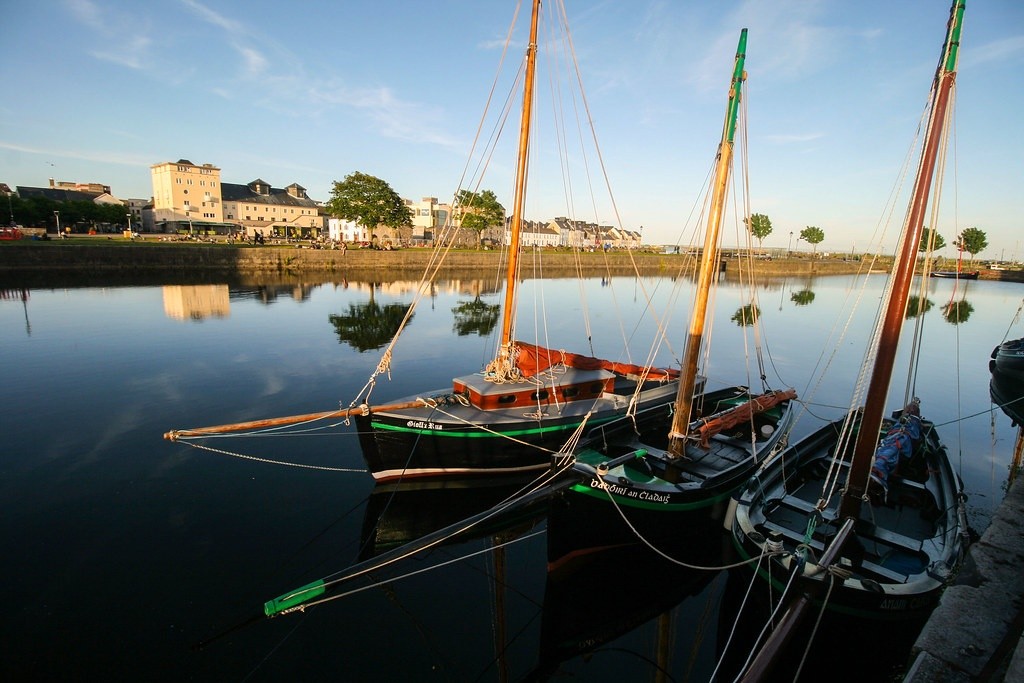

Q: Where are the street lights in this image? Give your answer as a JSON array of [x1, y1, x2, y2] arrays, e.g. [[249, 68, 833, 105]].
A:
[[126, 213, 132, 232], [639, 226, 643, 249], [54, 211, 61, 237], [789, 231, 794, 253]]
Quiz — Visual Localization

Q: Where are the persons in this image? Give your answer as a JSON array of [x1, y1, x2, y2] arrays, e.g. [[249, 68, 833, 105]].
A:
[[32, 233, 39, 241], [253, 229, 264, 246], [228, 232, 230, 238], [292, 230, 297, 238], [331, 242, 347, 255]]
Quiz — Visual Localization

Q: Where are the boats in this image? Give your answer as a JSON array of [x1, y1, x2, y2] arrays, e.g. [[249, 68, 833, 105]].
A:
[[989, 375, 1024, 435], [993, 295, 1024, 382], [930, 267, 979, 280]]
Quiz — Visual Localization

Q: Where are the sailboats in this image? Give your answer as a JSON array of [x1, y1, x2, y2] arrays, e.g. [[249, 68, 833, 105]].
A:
[[352, 1, 716, 453], [725, 0, 983, 616], [546, 23, 800, 521]]
